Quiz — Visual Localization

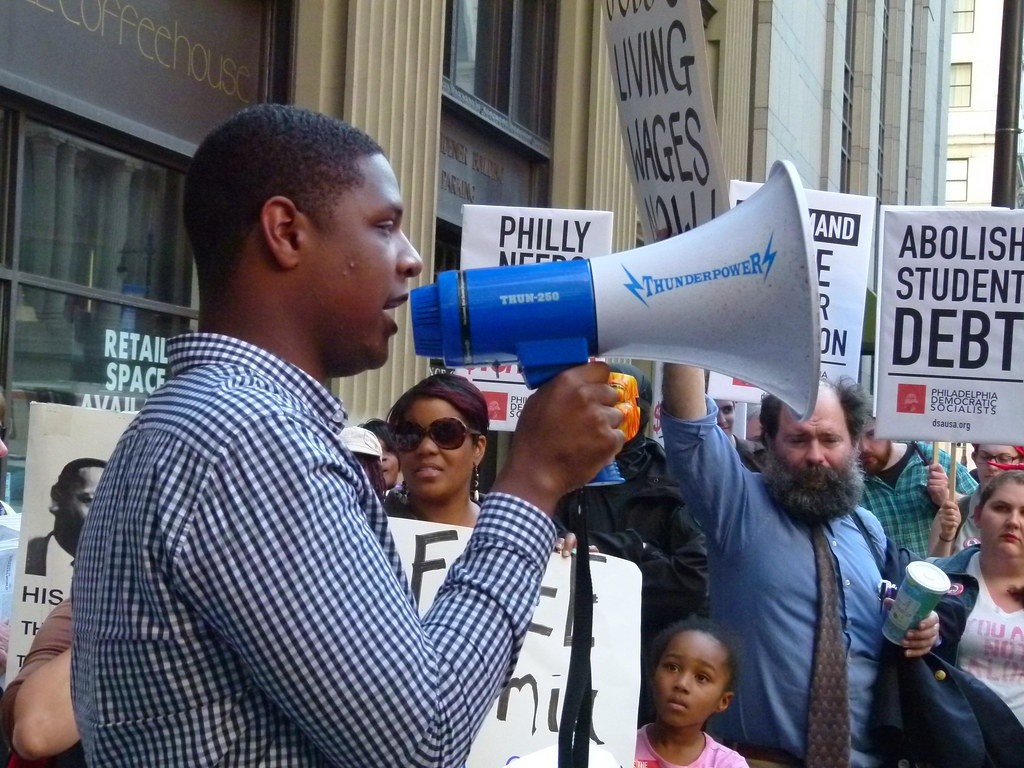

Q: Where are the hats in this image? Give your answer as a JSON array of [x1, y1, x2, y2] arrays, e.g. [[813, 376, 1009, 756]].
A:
[[339, 425, 384, 461]]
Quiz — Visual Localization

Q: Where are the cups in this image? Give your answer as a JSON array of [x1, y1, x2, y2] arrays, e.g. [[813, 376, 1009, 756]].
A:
[[881, 561, 951, 645]]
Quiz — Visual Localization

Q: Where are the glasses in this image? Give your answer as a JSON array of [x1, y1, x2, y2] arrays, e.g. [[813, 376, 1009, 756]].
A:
[[971, 451, 1021, 471], [391, 416, 483, 452]]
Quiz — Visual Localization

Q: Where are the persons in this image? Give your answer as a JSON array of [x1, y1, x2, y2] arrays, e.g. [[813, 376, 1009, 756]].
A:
[[25, 459, 108, 579], [0, 390, 88, 768], [554, 361, 1024, 768], [70, 104, 625, 768]]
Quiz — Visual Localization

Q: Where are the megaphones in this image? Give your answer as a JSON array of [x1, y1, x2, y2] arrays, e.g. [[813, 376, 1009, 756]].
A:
[[409, 158, 821, 489]]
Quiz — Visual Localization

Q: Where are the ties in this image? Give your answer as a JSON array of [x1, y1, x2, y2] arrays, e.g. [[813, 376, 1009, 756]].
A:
[[806, 516, 855, 766]]
